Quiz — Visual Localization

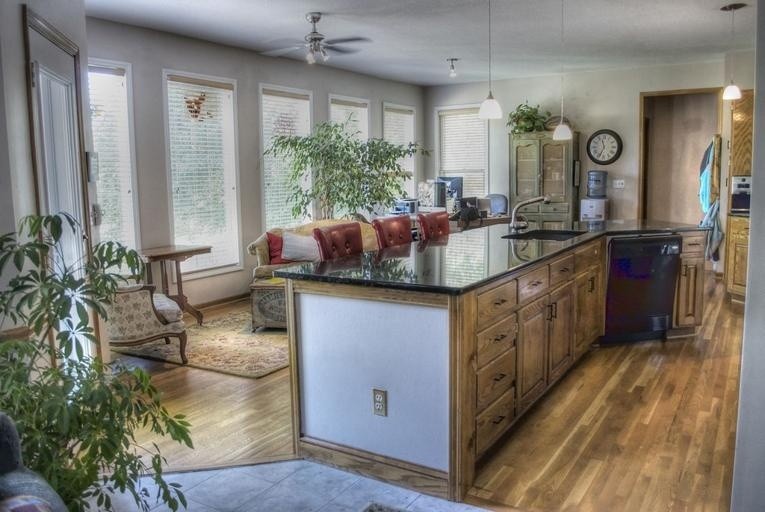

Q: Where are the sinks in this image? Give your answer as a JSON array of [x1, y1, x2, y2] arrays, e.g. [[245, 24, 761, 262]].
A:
[[500, 225, 589, 243]]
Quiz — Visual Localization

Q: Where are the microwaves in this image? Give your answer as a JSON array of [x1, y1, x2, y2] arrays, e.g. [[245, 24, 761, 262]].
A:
[[730, 176, 752, 217]]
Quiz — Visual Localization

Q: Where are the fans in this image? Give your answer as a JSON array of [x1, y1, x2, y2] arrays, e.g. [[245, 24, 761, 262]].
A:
[[256, 11, 374, 58]]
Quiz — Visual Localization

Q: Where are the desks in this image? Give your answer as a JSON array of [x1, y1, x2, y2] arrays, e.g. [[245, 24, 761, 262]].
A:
[[272, 219, 714, 503], [139, 244, 210, 325]]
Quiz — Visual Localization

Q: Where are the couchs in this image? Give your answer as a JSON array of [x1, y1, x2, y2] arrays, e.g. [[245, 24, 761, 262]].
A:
[[107, 285, 188, 364], [246, 219, 380, 333], [0, 412, 69, 512]]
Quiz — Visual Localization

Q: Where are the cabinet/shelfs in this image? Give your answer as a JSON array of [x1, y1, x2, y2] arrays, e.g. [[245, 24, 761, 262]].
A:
[[508, 130, 580, 222], [725, 215, 750, 297]]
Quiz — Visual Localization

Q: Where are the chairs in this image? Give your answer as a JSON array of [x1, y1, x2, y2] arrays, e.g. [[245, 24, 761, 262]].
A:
[[312, 222, 364, 260], [371, 215, 412, 249], [485, 193, 507, 215], [417, 212, 450, 241]]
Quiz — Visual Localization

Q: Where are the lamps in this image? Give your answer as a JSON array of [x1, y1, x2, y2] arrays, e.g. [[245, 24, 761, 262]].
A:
[[303, 41, 330, 64], [478, 0, 502, 119], [553, 1, 573, 140], [723, 8, 741, 99], [447, 59, 457, 78]]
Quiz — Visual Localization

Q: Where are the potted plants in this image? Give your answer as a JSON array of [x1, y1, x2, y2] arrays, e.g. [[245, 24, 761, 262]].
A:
[[505, 96, 551, 134]]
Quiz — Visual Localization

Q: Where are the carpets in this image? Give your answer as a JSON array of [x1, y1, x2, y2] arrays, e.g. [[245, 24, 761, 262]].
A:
[[108, 296, 289, 380]]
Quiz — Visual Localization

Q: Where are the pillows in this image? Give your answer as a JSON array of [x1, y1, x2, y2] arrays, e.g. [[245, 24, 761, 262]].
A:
[[280, 231, 321, 262], [266, 231, 284, 264]]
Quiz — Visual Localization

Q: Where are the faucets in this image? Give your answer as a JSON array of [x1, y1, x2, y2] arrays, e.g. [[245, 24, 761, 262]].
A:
[[509, 196, 552, 230], [510, 240, 532, 262]]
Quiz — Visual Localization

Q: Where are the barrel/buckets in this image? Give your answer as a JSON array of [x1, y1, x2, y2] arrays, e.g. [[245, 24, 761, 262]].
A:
[[588, 220, 606, 232], [587, 170, 608, 197]]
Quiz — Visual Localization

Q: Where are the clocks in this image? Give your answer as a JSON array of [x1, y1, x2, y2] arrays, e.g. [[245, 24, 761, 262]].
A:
[[586, 129, 623, 164]]
